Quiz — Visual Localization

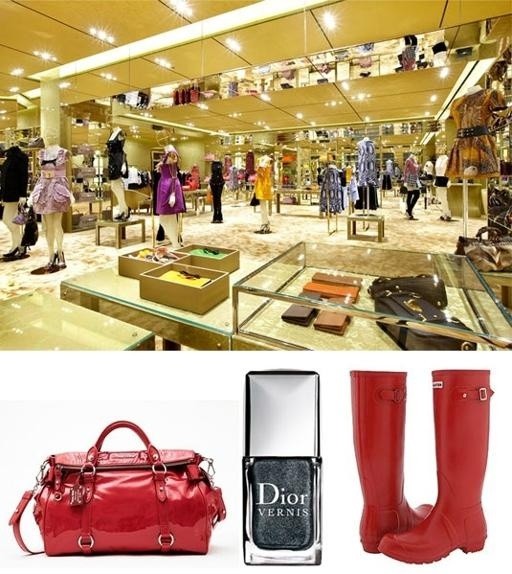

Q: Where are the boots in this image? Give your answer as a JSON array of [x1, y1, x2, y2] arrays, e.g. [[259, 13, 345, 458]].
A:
[[378, 370, 494, 563], [350, 370, 432, 553]]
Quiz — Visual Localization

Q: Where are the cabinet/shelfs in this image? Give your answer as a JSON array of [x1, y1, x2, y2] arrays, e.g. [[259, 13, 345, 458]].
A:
[[59, 102, 112, 233]]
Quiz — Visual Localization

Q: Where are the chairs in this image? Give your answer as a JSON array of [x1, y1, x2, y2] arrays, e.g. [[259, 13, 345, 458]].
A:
[[123, 188, 150, 215]]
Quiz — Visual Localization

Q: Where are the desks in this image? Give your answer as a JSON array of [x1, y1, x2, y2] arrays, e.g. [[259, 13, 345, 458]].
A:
[[181, 188, 207, 216], [273, 188, 325, 218]]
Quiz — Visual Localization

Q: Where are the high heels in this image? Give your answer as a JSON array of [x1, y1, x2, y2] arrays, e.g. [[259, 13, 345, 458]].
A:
[[114, 211, 124, 219], [15, 245, 30, 257], [3, 247, 18, 257], [56, 251, 65, 267], [123, 208, 131, 219], [260, 224, 266, 234], [266, 223, 270, 231], [43, 254, 57, 270]]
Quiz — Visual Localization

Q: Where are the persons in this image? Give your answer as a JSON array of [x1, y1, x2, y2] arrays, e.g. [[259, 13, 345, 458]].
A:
[[112, 30, 431, 114], [448, 81, 507, 185], [1, 122, 445, 274]]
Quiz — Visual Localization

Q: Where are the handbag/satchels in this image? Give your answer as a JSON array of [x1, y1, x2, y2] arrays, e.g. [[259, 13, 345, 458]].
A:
[[367, 274, 447, 309], [250, 193, 259, 206], [79, 188, 96, 202], [400, 187, 407, 194], [27, 137, 45, 148], [488, 185, 512, 226], [375, 292, 447, 323], [376, 316, 477, 350], [456, 227, 512, 272], [72, 209, 83, 224], [13, 203, 27, 225], [80, 167, 96, 177], [21, 220, 38, 246], [34, 421, 226, 556], [435, 176, 448, 187], [79, 211, 98, 228], [102, 206, 111, 220]]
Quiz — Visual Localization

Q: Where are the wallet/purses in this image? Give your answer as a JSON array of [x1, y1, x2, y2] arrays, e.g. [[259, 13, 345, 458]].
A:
[[314, 298, 352, 335], [303, 282, 359, 304], [311, 273, 363, 289], [186, 249, 227, 259], [157, 271, 211, 288], [282, 293, 321, 326]]
